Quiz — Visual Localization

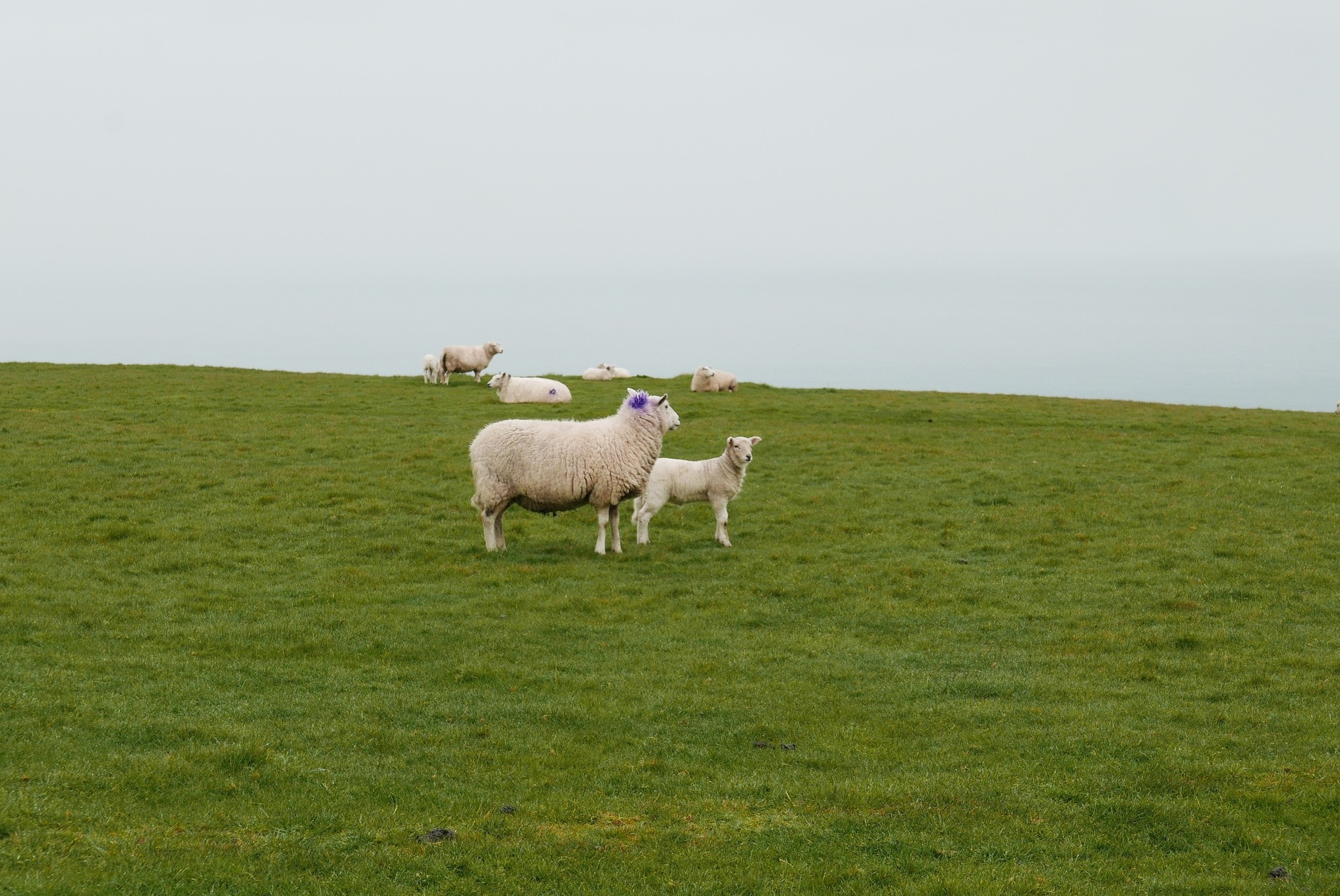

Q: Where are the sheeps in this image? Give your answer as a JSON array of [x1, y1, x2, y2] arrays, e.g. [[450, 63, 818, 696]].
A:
[[631, 436, 762, 547], [440, 340, 503, 385], [691, 365, 739, 392], [582, 362, 632, 380], [469, 388, 681, 556], [423, 354, 442, 384], [487, 370, 573, 404]]
